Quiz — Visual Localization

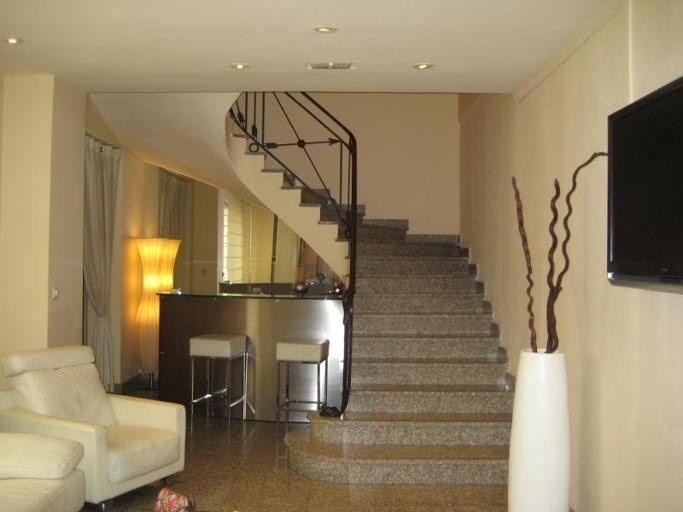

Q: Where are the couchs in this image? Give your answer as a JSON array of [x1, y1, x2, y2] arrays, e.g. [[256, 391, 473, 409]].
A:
[[0, 430, 86, 510]]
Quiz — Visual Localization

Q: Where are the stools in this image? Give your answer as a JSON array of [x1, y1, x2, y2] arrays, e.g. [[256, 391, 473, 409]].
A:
[[186, 332, 252, 427], [273, 336, 332, 428]]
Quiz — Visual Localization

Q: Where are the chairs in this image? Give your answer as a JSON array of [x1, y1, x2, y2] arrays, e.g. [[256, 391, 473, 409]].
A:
[[1, 344, 184, 512], [154, 485, 196, 511]]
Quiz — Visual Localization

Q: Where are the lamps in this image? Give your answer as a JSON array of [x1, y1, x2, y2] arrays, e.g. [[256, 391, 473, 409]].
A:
[[134, 236, 182, 392]]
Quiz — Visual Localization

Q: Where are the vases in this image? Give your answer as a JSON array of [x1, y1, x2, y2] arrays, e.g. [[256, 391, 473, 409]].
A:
[[506, 347, 572, 512]]
